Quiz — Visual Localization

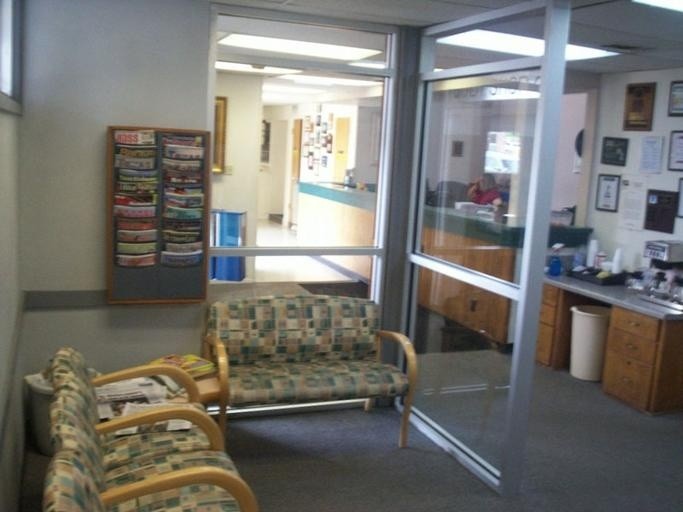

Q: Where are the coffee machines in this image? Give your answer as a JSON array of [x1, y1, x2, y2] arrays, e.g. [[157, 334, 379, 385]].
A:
[[639, 239, 683, 312]]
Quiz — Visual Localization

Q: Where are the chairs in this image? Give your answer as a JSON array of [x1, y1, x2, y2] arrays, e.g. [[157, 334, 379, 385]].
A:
[[44, 386, 232, 471], [43, 347, 214, 442], [42, 447, 259, 511]]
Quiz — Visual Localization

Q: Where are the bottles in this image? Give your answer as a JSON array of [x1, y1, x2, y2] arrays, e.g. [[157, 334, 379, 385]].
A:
[[570, 243, 585, 268], [586, 239, 598, 267], [549, 256, 561, 276], [612, 247, 624, 274]]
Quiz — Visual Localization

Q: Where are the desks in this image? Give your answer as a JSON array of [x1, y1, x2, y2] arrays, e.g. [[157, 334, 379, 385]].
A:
[[534, 268, 682, 415]]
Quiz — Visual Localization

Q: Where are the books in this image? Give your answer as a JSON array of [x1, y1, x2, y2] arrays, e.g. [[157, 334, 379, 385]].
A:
[[90, 352, 216, 437], [111, 125, 204, 267]]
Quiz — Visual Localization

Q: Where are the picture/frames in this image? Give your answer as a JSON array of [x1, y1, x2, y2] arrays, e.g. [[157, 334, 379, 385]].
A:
[[588, 77, 682, 235], [299, 102, 334, 172], [212, 96, 229, 175]]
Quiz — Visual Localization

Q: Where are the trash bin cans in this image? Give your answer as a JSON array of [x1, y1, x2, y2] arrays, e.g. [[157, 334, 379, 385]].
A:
[[570, 305, 612, 382], [23, 366, 101, 456]]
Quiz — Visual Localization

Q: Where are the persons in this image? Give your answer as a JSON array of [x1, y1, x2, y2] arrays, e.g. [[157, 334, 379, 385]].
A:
[[467, 174, 502, 208]]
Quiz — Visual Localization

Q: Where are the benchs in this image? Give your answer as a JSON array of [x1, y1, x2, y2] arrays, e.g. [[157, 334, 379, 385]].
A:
[[198, 292, 418, 451]]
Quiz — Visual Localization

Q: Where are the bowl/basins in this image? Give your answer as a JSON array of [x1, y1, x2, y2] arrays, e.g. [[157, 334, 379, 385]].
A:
[[550, 210, 573, 226]]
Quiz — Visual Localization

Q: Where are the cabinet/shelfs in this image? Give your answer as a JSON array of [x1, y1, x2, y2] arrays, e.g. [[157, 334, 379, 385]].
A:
[[100, 122, 211, 305]]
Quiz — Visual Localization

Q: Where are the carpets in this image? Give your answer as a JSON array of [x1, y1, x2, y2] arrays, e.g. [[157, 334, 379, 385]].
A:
[[295, 281, 498, 356]]
